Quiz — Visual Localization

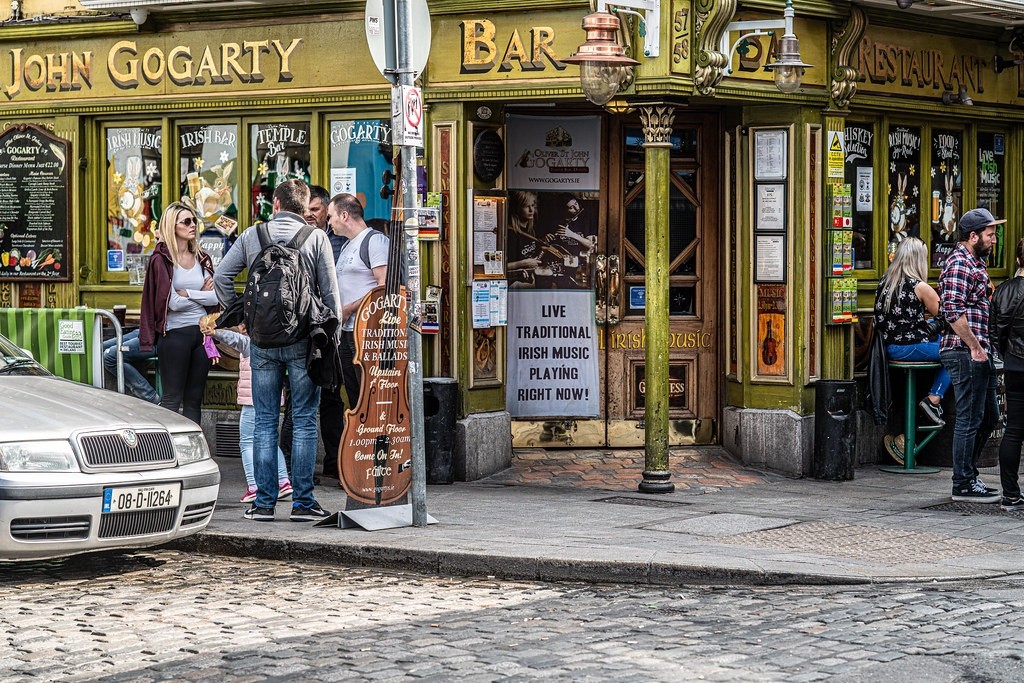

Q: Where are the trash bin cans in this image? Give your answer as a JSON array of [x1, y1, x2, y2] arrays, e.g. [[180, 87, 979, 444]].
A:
[[422, 377, 457, 485], [814, 379, 856, 481]]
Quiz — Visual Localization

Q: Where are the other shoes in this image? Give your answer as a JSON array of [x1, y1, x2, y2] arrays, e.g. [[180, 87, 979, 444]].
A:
[[313, 477, 320, 484], [151, 393, 162, 405], [323, 468, 339, 478]]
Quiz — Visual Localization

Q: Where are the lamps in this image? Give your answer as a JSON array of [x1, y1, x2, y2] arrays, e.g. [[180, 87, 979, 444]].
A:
[[603, 97, 635, 116], [941, 83, 973, 107], [723, 1, 814, 93], [560, 0, 660, 105]]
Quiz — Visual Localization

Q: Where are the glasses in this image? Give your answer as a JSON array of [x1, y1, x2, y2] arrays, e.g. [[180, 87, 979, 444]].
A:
[[176, 217, 197, 227]]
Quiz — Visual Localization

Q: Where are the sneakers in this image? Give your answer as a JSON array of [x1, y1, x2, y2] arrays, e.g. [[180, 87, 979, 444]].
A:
[[971, 478, 1000, 494], [951, 480, 1001, 502], [1001, 492, 1024, 510], [919, 397, 947, 424], [243, 506, 275, 520], [277, 482, 293, 499], [290, 501, 332, 521], [240, 485, 258, 502]]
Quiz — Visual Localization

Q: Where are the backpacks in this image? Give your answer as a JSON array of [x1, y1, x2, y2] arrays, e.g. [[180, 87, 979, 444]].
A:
[[243, 221, 317, 349], [360, 230, 406, 286]]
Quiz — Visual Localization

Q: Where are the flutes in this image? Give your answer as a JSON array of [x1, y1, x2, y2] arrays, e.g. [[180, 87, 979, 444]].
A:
[[554, 208, 585, 237]]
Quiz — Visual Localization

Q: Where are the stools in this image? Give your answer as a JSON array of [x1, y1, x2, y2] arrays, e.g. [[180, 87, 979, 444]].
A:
[[878, 361, 942, 474]]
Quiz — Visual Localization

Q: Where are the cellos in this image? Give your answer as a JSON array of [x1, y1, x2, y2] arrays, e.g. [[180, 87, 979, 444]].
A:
[[335, 150, 414, 507]]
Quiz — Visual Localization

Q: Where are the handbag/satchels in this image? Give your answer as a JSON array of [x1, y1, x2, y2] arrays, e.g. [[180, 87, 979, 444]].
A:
[[863, 328, 897, 425]]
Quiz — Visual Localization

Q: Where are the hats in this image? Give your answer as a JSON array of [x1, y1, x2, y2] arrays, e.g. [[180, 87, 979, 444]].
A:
[[958, 207, 1008, 232], [883, 434, 917, 466]]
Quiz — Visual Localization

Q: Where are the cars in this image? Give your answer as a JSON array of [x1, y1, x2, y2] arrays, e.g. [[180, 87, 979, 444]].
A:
[[0, 334, 220, 564]]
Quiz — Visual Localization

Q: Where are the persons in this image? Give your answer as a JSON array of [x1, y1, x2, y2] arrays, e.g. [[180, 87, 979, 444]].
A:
[[139, 201, 220, 427], [873, 236, 952, 425], [279, 185, 348, 484], [104, 328, 162, 405], [506, 191, 594, 288], [212, 179, 343, 521], [201, 322, 293, 502], [989, 237, 1024, 511], [938, 208, 1007, 503], [328, 193, 389, 411]]
